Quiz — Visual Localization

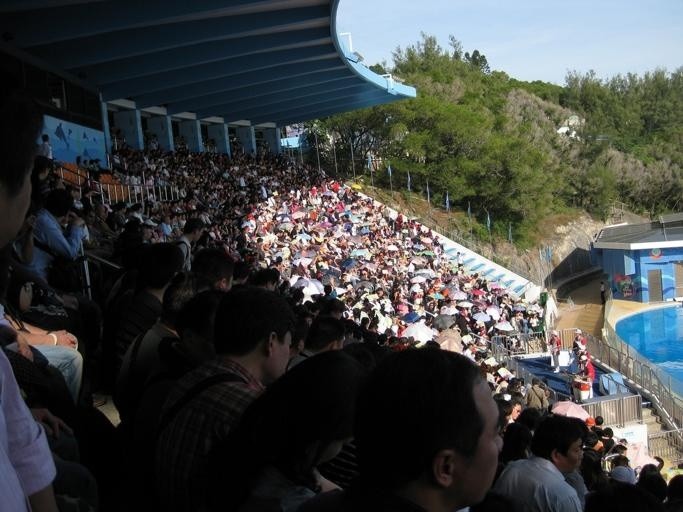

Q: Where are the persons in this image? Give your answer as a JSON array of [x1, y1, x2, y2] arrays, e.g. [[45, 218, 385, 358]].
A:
[[599, 281, 606, 304], [1, 55, 108, 512], [570, 329, 595, 397], [498, 393, 682, 511], [86, 133, 549, 512], [542, 331, 563, 375]]
